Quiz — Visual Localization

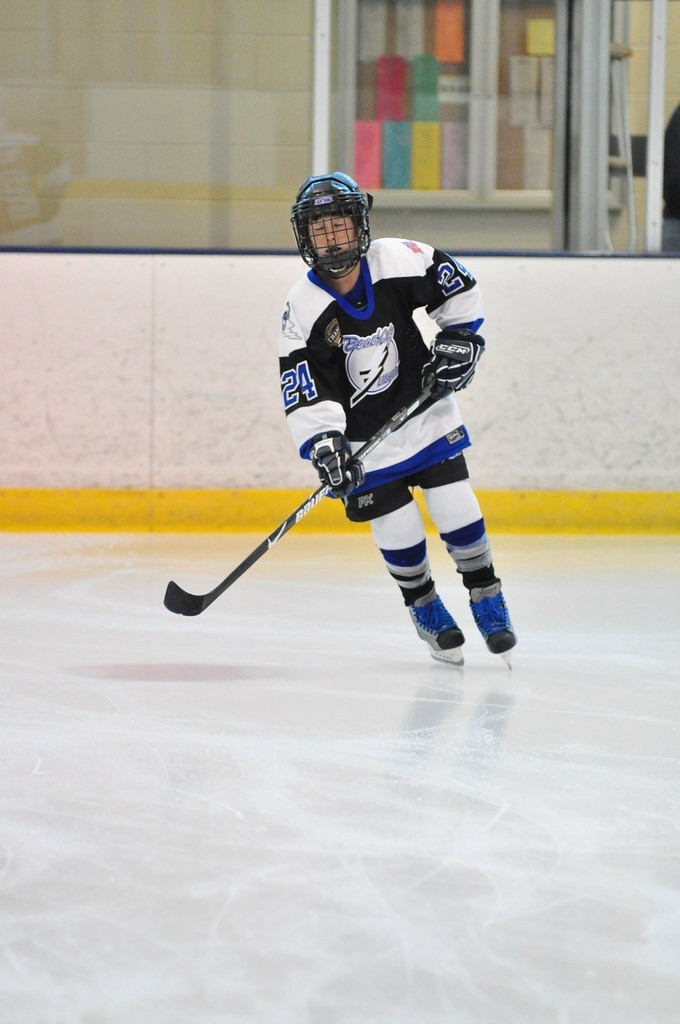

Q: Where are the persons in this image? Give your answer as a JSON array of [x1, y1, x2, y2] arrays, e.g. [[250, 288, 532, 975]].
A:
[[278, 171, 519, 655]]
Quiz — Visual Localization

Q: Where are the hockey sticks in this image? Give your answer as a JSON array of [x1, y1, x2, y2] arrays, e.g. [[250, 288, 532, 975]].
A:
[[161, 376, 439, 618]]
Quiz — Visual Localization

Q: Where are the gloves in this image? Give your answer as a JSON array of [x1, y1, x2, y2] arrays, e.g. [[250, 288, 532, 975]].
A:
[[421, 334, 485, 393], [308, 432, 365, 498]]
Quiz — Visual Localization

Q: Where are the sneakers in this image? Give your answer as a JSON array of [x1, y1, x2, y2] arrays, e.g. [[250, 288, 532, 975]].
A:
[[402, 581, 465, 666], [468, 577, 518, 671]]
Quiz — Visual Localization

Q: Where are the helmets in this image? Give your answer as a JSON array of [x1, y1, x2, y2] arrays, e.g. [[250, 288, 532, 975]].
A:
[[290, 171, 374, 279]]
